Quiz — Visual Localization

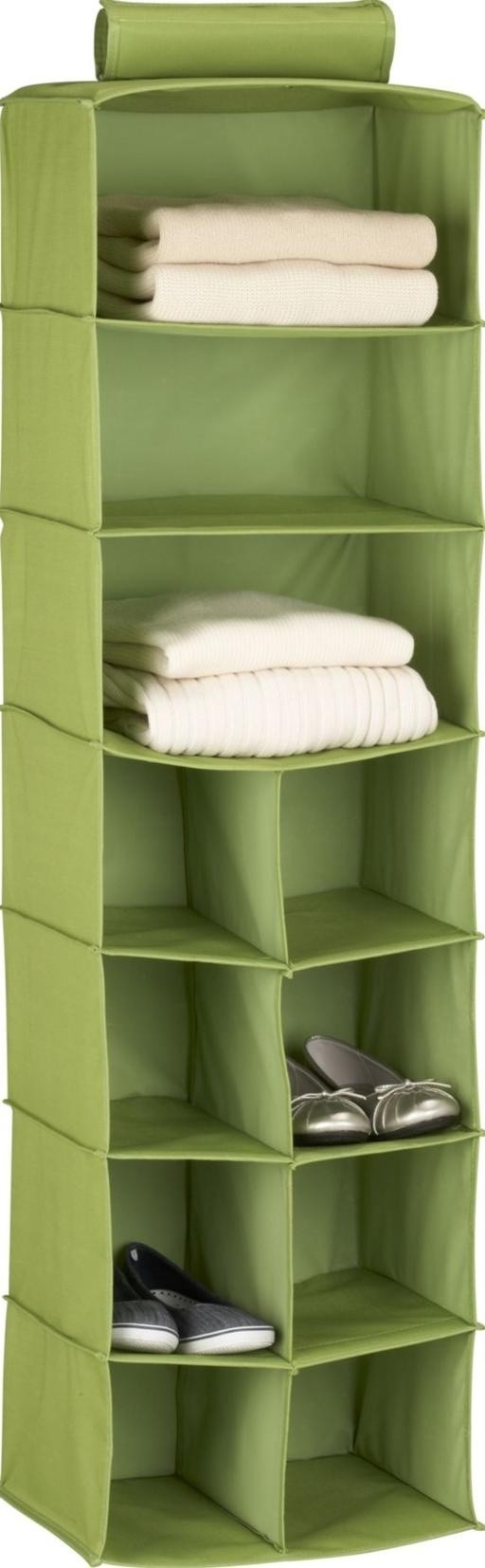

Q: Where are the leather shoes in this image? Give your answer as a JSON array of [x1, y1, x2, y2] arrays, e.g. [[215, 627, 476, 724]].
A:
[[286, 1035, 460, 1145]]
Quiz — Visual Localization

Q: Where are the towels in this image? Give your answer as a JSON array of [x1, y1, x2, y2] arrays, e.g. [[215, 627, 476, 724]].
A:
[[101, 261, 442, 329], [103, 663, 440, 761], [99, 590, 416, 684], [99, 191, 441, 272]]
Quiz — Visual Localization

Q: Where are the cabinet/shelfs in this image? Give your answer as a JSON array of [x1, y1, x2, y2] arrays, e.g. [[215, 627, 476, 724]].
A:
[[1, 1, 485, 1568]]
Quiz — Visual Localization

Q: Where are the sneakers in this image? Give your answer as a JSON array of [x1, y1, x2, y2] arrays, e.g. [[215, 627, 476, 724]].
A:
[[113, 1243, 276, 1354]]
